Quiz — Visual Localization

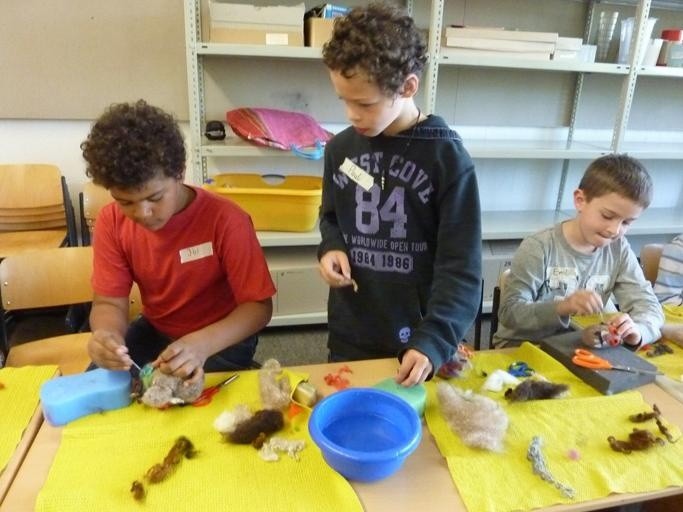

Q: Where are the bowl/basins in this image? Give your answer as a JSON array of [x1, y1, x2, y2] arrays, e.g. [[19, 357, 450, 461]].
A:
[[308, 387, 423, 482]]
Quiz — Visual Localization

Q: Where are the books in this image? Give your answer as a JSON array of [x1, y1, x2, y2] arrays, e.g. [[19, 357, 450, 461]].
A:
[[441, 24, 598, 66], [198, 0, 305, 47]]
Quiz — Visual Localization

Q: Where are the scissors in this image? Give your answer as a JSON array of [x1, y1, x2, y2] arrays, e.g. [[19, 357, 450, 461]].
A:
[[572, 349, 637, 373], [189, 375, 238, 406], [507, 362, 535, 376]]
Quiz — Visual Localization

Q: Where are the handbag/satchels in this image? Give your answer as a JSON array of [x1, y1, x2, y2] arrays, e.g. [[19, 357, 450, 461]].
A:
[[227, 108, 334, 160]]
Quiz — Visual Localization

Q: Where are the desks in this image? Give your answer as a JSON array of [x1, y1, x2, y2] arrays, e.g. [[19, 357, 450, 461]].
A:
[[1, 337, 682, 511]]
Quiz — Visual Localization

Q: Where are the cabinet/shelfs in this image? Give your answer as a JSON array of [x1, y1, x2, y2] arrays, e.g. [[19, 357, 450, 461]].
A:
[[183, 1, 683, 331]]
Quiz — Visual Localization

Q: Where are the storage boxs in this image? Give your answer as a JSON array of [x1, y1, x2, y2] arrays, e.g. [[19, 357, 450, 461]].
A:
[[210, 3, 305, 47], [201, 173, 323, 232], [478, 240, 523, 300], [305, 18, 335, 46], [263, 248, 330, 315]]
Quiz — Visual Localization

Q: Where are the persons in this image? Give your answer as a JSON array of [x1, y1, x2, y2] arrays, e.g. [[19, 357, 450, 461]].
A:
[[652, 232, 683, 349], [314, 1, 484, 389], [79, 99, 280, 392], [489, 150, 665, 353]]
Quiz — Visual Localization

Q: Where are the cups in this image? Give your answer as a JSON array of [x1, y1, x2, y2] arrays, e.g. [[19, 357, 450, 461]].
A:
[[595, 10, 621, 62]]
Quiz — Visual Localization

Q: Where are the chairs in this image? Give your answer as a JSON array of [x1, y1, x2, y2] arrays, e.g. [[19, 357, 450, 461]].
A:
[[637, 244, 664, 288], [474, 279, 484, 350], [489, 268, 511, 349], [0, 164, 146, 378]]
